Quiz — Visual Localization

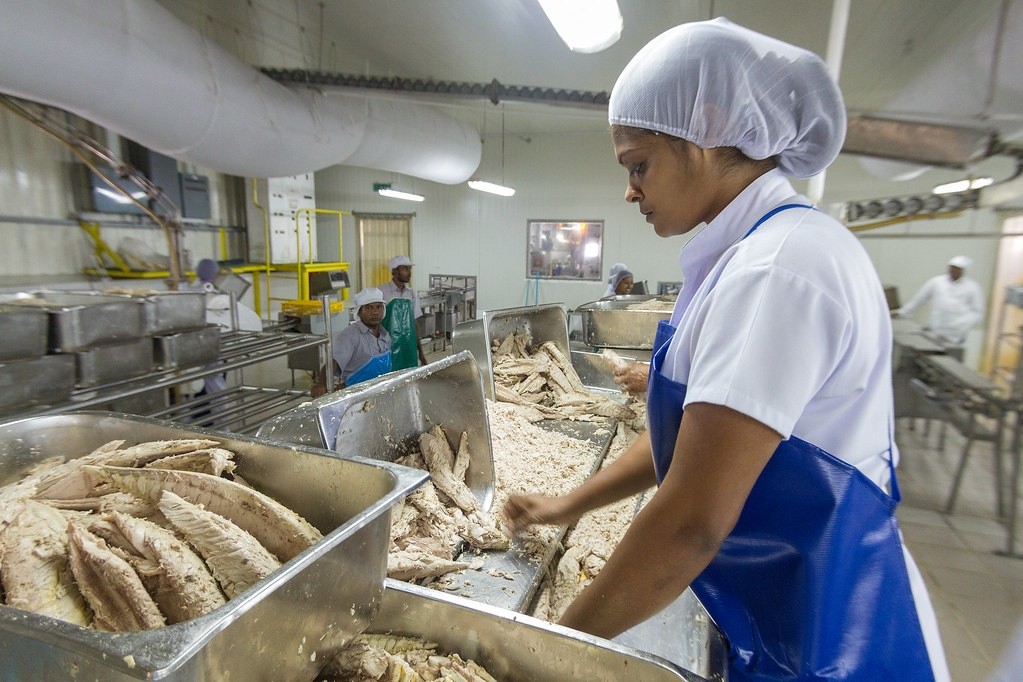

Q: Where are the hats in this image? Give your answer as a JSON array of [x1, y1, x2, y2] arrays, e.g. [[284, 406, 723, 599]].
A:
[[608, 17, 846, 179], [608, 263, 628, 284], [389, 255, 414, 274], [610, 269, 633, 293], [353, 287, 387, 321], [947, 255, 970, 268]]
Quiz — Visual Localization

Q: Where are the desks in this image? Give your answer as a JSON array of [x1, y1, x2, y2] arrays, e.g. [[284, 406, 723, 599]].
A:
[[889, 317, 1023, 524]]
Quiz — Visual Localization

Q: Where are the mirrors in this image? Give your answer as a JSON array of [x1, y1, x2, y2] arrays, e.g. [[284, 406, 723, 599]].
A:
[[525, 219, 604, 282]]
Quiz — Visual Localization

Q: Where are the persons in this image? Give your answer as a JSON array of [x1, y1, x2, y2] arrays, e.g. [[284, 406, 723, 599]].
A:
[[890, 255, 986, 392], [604, 263, 634, 298], [613, 361, 649, 401], [504, 16, 954, 682], [311, 286, 392, 397], [378, 255, 428, 371]]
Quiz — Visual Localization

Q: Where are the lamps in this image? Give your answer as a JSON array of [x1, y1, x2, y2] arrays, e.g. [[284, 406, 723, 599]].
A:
[[378, 172, 425, 202], [468, 101, 516, 197]]
